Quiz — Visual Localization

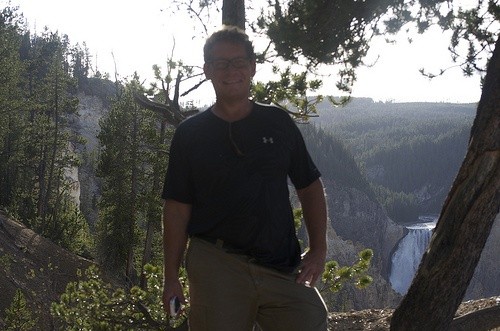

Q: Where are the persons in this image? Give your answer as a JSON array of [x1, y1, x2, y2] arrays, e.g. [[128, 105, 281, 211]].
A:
[[161, 28, 329, 330]]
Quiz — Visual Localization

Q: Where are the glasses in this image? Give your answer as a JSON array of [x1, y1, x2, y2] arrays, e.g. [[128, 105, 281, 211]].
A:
[[205, 53, 255, 71]]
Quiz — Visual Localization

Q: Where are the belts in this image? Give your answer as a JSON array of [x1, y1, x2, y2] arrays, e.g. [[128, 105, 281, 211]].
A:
[[192, 231, 253, 257]]
